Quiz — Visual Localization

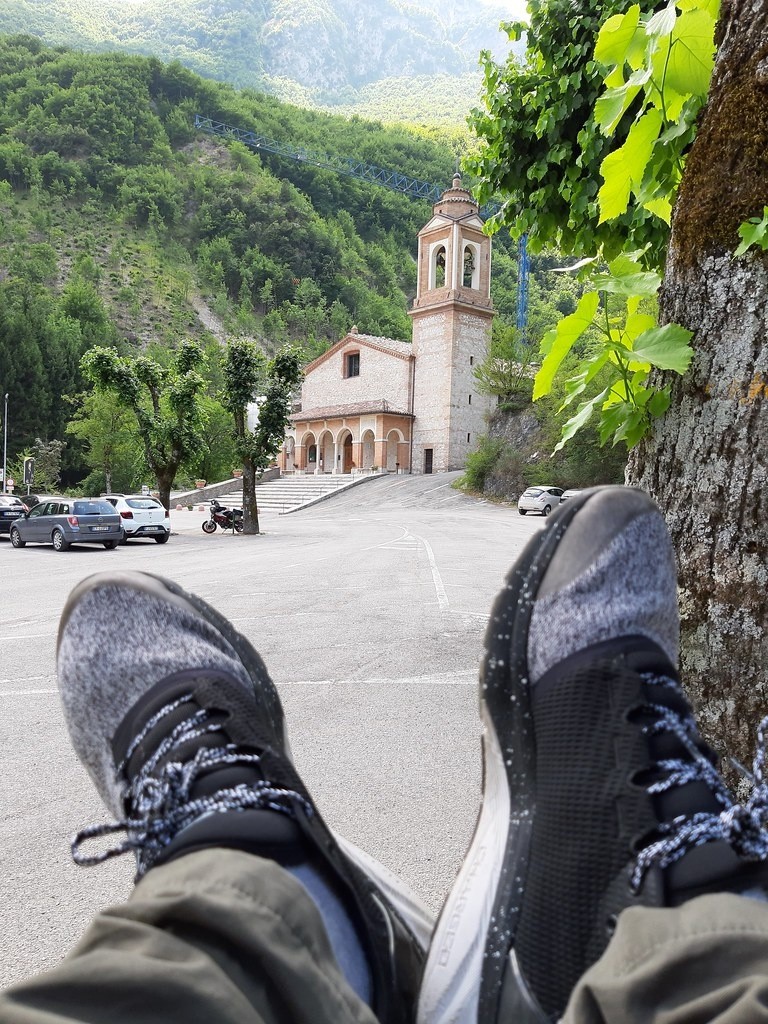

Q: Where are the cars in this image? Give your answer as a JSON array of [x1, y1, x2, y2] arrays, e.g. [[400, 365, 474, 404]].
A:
[[518, 485, 565, 516], [10, 497, 124, 551], [0, 494, 29, 535], [19, 495, 71, 515], [559, 488, 581, 506]]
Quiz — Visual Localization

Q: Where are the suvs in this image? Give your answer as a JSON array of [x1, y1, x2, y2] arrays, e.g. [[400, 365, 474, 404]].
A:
[[99, 493, 172, 545]]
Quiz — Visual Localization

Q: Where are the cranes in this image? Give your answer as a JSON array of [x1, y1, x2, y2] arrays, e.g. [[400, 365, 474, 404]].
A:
[[193, 114, 529, 353]]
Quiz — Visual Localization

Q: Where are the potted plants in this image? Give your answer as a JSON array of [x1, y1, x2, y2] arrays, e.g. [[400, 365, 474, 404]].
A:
[[232, 469, 244, 478], [187, 504, 193, 512], [195, 479, 206, 489]]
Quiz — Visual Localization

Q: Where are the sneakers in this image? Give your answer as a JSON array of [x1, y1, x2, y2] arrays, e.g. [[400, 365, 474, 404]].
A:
[[55, 568, 436, 1024], [417, 486, 768, 1024]]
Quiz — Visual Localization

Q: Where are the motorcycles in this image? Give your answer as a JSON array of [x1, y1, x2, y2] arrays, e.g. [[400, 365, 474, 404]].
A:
[[202, 499, 243, 534]]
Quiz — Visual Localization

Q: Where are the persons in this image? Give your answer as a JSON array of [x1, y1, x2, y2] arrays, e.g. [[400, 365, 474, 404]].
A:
[[0, 485, 768, 1024]]
